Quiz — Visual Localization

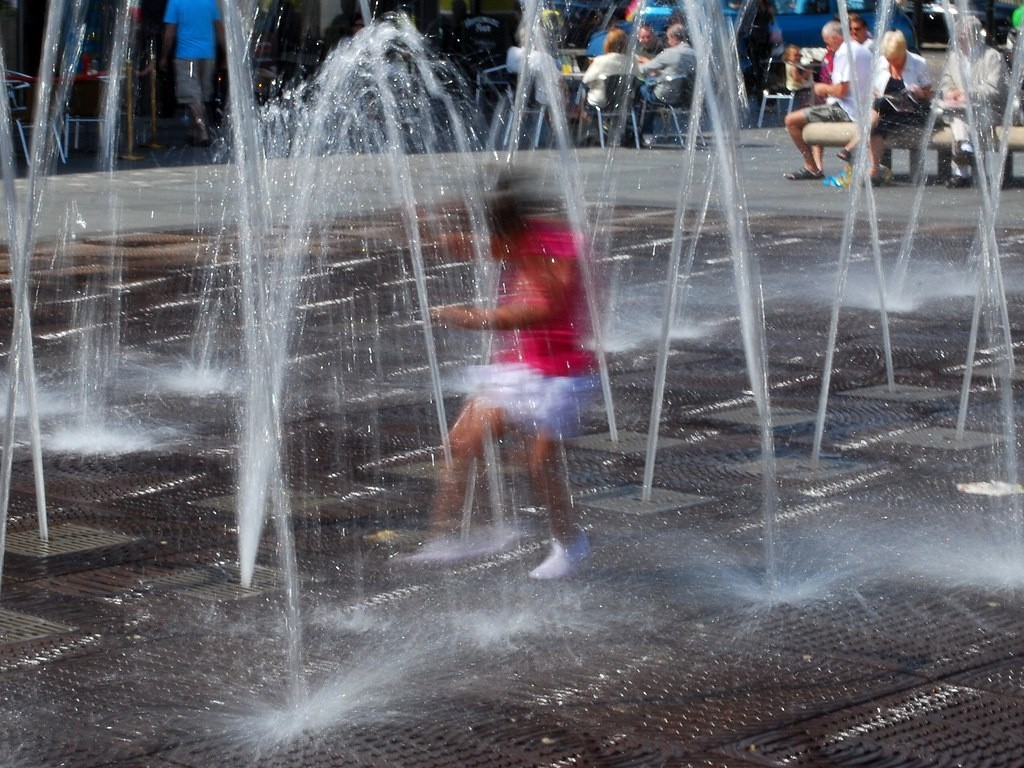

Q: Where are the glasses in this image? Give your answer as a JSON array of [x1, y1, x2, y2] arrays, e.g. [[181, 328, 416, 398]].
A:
[[849, 26, 864, 31]]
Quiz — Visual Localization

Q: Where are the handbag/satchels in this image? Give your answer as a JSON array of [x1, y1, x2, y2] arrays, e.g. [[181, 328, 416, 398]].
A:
[[885, 90, 945, 128]]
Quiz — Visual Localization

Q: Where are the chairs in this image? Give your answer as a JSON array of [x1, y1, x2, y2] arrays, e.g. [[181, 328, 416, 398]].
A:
[[638, 64, 707, 148], [584, 72, 641, 150], [65, 77, 109, 156], [12, 82, 67, 167], [757, 60, 811, 129], [502, 71, 547, 149]]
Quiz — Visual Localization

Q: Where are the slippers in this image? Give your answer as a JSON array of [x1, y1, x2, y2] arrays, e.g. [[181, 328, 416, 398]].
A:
[[786, 167, 824, 180], [837, 147, 851, 161], [860, 177, 880, 188]]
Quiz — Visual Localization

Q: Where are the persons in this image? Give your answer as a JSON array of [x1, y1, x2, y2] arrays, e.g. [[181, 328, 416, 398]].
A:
[[574, 11, 695, 144], [542, 3, 564, 34], [939, 16, 1007, 187], [263, 0, 471, 57], [507, 26, 568, 110], [406, 175, 591, 580], [162, 0, 224, 144], [728, 0, 776, 98], [838, 30, 932, 185], [127, 25, 157, 76], [784, 15, 874, 180]]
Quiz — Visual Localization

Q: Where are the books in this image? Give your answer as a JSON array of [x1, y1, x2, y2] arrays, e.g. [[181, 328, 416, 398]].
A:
[[878, 90, 920, 112]]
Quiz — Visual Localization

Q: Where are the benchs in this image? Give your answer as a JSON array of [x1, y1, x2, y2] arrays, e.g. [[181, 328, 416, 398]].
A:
[[804, 121, 1024, 187]]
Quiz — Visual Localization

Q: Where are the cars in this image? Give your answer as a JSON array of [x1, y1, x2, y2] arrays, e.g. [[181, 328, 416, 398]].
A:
[[550, 1, 1021, 71]]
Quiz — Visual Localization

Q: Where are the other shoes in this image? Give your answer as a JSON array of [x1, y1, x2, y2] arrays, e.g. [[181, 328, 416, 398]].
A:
[[951, 150, 977, 165], [527, 526, 591, 579], [642, 133, 655, 146], [945, 175, 973, 188]]
